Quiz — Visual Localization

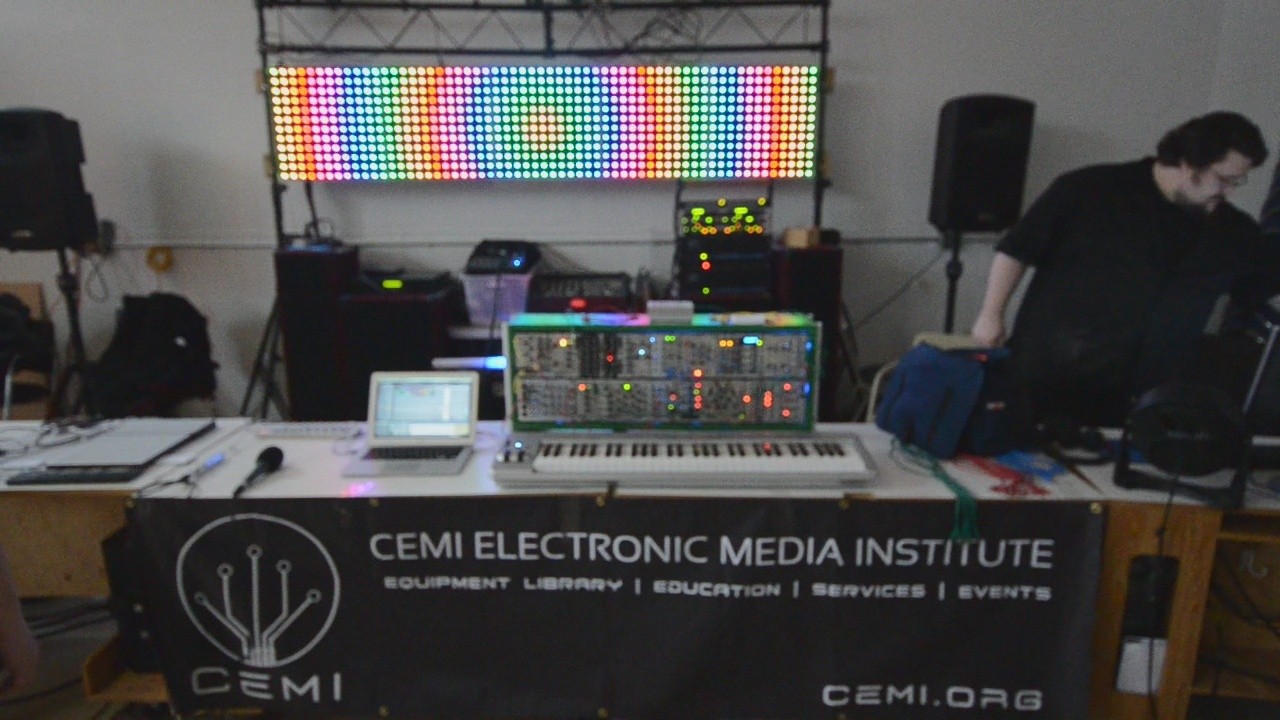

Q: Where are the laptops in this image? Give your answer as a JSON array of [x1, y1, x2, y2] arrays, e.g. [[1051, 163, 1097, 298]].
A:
[[343, 369, 482, 479]]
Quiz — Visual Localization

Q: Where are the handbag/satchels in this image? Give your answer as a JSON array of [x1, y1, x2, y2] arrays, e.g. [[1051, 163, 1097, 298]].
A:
[[876, 341, 1016, 458]]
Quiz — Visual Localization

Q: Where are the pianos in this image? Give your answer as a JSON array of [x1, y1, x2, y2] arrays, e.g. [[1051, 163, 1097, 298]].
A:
[[491, 426, 882, 485]]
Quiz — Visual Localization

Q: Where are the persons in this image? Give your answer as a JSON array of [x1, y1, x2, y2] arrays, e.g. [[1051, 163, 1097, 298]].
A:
[[970, 109, 1280, 450]]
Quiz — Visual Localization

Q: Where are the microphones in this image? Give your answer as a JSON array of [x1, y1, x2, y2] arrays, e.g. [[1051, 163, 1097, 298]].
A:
[[232, 448, 283, 494]]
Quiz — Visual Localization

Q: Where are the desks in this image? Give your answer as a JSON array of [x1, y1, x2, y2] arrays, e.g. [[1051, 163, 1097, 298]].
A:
[[0, 418, 1280, 720]]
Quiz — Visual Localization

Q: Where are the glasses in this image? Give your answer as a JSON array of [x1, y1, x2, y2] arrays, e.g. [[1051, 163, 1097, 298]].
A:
[[1206, 166, 1248, 188]]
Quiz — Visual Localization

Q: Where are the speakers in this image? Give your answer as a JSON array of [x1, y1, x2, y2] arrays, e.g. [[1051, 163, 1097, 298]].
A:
[[927, 92, 1033, 232], [331, 279, 459, 427], [1, 108, 97, 253]]
